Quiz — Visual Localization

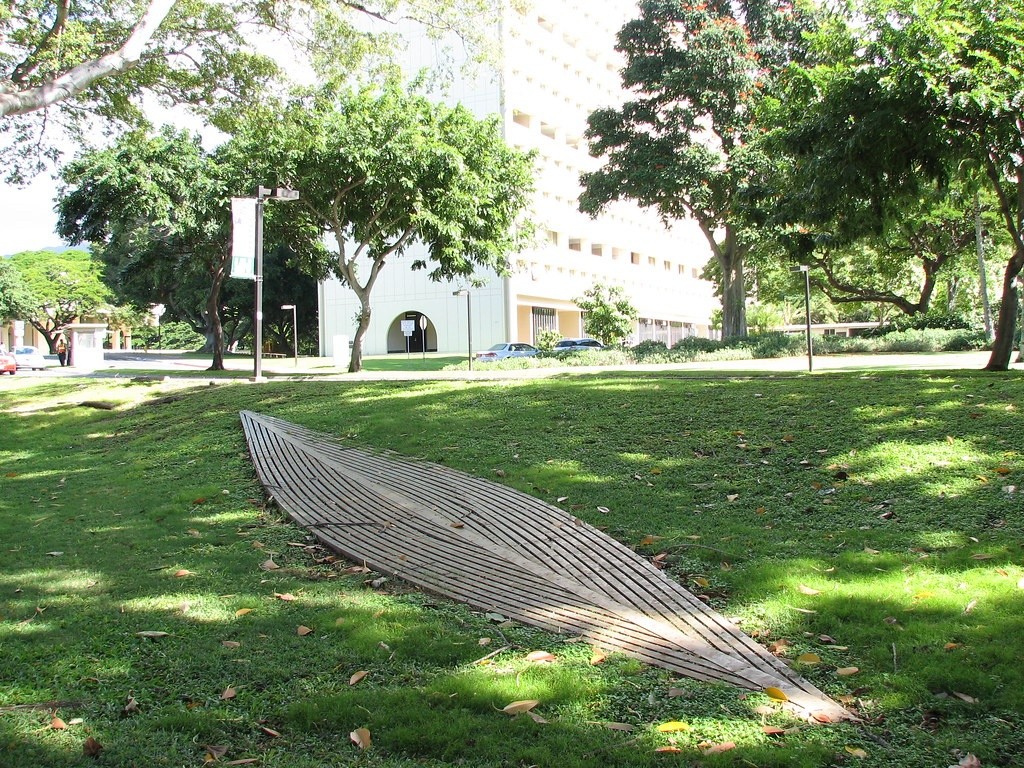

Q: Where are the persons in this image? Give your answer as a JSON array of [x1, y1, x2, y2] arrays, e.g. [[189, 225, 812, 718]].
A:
[[0, 342, 7, 356], [57, 339, 66, 366]]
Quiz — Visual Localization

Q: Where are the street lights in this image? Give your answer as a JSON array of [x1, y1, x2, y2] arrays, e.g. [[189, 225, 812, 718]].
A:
[[789, 264, 813, 372], [281, 304, 298, 367], [452, 287, 472, 371]]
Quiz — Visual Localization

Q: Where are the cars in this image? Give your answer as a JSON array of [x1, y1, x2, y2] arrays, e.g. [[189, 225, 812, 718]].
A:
[[552, 338, 605, 352], [10, 345, 46, 372], [0, 349, 16, 375], [476, 342, 542, 363]]
